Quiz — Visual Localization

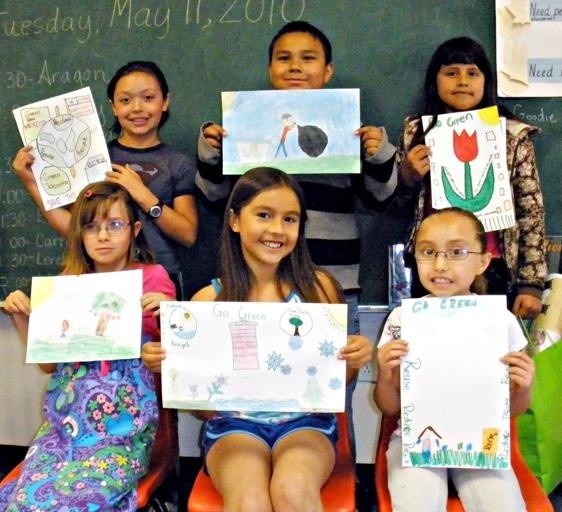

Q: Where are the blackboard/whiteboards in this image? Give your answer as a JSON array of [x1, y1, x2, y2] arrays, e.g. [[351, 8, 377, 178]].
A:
[[497, 96, 562, 245], [0, 0, 498, 314]]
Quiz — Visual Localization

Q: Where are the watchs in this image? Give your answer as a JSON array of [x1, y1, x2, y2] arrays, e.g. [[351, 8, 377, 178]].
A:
[[146, 198, 164, 220]]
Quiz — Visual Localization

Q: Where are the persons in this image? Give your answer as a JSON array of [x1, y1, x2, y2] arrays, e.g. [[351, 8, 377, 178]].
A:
[[13, 60, 199, 301], [141, 167, 373, 512], [397, 37, 548, 332], [0, 182, 177, 512], [194, 21, 399, 335], [374, 207, 536, 512]]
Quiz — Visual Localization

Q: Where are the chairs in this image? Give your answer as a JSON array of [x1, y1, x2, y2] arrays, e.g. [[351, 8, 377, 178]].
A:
[[375, 415, 555, 512], [188, 411, 356, 512], [0, 342, 175, 512]]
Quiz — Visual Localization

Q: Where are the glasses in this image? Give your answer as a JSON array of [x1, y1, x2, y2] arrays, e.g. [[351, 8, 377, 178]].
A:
[[84, 221, 132, 233], [414, 246, 484, 259]]
